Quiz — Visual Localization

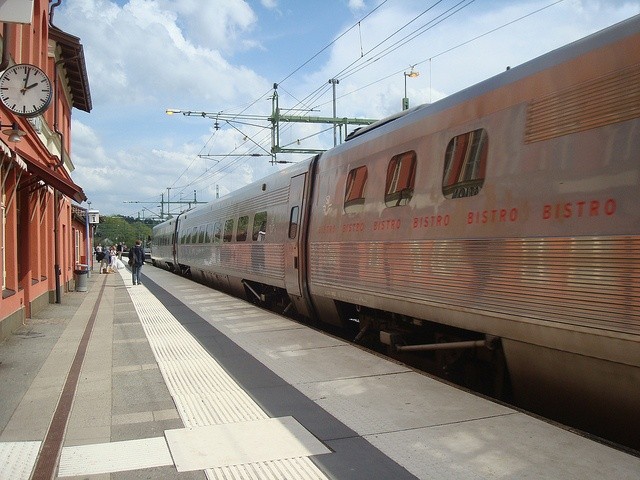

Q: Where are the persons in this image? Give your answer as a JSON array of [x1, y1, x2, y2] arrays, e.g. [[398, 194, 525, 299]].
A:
[[95, 242, 127, 274], [128, 240, 146, 286]]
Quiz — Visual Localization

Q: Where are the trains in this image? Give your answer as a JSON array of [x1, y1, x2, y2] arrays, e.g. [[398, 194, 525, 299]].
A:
[[149, 11, 640, 457]]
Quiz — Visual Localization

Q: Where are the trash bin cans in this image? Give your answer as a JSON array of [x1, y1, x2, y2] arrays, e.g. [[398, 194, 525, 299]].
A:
[[75, 270, 88, 291]]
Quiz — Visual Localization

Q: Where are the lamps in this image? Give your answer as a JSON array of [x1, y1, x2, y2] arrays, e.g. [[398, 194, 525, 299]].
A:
[[0, 121, 27, 142]]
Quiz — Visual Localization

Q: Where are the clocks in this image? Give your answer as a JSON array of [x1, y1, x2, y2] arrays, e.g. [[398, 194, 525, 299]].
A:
[[0, 64, 52, 117]]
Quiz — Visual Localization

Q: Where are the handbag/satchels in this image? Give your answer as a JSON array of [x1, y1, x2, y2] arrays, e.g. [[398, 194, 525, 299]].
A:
[[128, 248, 136, 267]]
